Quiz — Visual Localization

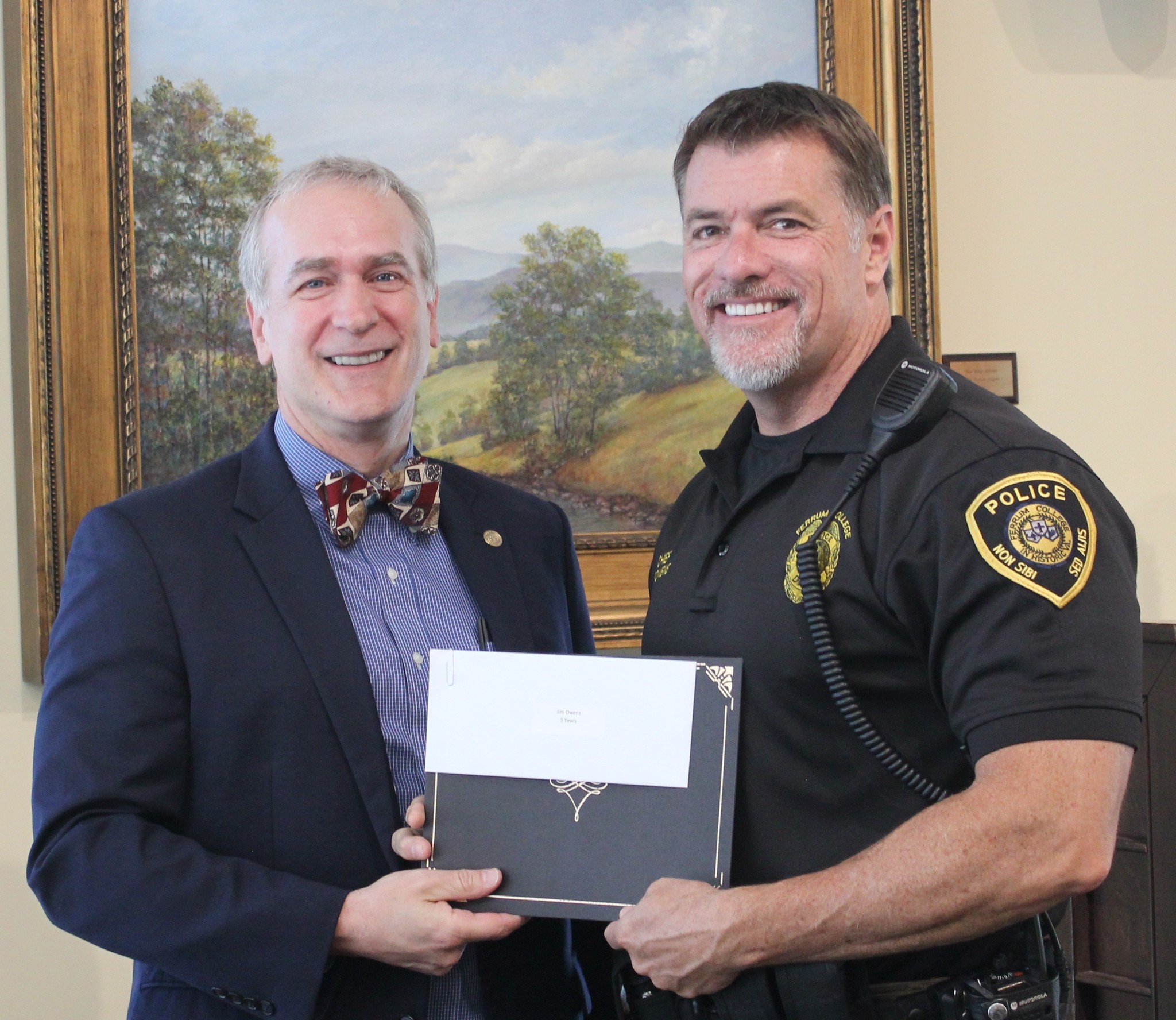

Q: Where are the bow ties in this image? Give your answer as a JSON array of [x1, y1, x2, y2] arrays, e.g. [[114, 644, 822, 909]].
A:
[[315, 456, 443, 549]]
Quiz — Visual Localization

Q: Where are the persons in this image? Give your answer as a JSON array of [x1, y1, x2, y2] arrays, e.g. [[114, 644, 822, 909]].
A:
[[24, 159, 604, 1020], [393, 81, 1142, 1020]]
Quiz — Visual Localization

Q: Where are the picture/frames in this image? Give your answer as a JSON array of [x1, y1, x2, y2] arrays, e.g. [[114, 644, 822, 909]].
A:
[[3, 0, 938, 686]]
